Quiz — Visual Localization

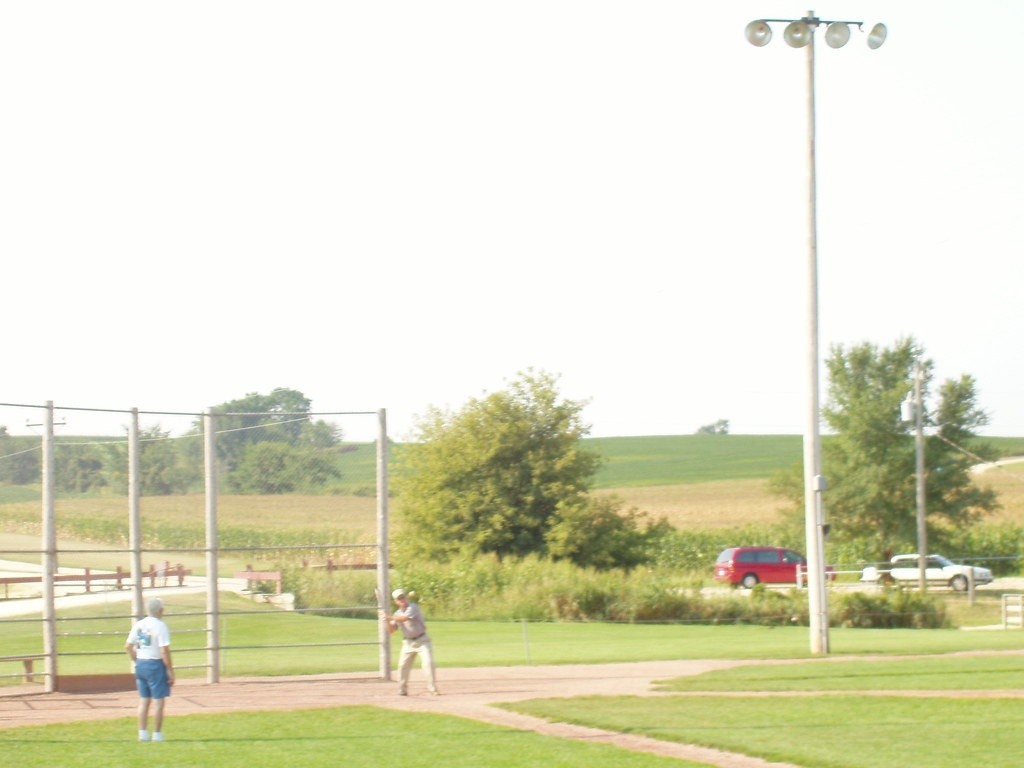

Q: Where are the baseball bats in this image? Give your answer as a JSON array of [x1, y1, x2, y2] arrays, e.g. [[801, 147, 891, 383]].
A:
[[374, 588, 394, 634]]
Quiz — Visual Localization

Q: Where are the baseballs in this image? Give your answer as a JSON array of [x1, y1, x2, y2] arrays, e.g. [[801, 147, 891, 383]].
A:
[[408, 590, 416, 597]]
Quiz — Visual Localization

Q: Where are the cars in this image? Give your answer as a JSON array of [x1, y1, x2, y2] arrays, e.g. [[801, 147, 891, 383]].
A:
[[859, 555, 994, 591]]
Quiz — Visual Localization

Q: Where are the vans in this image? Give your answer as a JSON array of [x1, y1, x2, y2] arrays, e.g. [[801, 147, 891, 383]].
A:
[[714, 547, 836, 589]]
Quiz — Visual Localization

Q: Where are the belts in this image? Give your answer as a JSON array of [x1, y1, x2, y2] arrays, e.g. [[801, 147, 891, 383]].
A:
[[405, 632, 424, 640]]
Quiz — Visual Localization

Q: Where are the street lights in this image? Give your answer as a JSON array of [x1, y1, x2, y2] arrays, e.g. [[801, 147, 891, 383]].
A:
[[742, 11, 886, 662]]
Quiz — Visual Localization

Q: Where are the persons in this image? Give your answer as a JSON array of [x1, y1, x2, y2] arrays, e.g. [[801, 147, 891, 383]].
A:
[[378, 589, 441, 698], [124, 599, 175, 741]]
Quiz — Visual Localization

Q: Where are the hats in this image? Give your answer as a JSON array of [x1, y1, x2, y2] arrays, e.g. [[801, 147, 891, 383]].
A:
[[392, 588, 405, 601]]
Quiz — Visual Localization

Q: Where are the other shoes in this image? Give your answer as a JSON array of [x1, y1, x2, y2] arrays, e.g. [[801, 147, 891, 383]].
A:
[[152, 732, 163, 741], [397, 693, 407, 697], [139, 730, 150, 740], [430, 690, 439, 696]]
